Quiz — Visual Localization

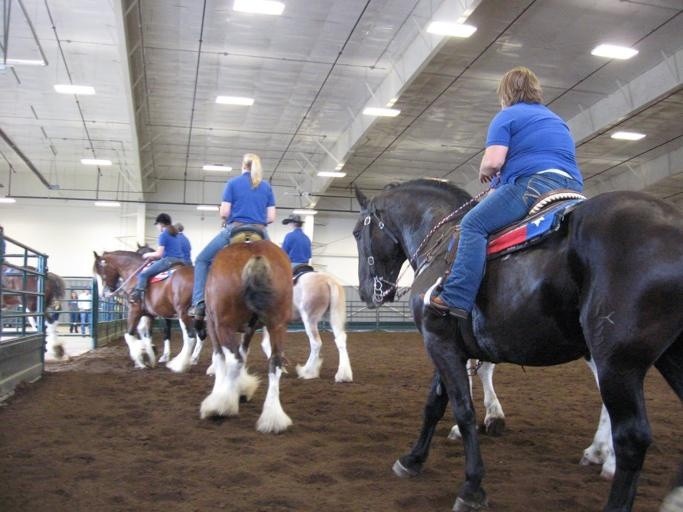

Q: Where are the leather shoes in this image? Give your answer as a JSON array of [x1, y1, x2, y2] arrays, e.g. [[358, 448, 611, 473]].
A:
[[187, 303, 205, 320], [419, 291, 469, 319]]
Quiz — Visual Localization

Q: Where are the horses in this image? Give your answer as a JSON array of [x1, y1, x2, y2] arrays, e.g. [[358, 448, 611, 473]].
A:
[[0, 260, 73, 363], [261, 270, 354, 385], [447, 355, 617, 481], [198, 239, 294, 433], [352, 176, 683, 512], [92, 242, 207, 373]]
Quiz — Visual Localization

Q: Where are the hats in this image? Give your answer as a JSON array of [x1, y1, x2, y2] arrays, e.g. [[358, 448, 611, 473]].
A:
[[154, 213, 171, 225], [174, 223, 184, 231], [282, 215, 304, 225]]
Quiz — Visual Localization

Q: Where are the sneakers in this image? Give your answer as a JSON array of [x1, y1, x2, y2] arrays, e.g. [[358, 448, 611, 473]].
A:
[[128, 290, 142, 297]]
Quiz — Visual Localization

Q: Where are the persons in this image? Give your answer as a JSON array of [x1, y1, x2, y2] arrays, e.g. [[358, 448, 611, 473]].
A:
[[127, 213, 184, 300], [419, 67, 583, 319], [186, 153, 276, 320], [173, 223, 192, 266], [67, 292, 79, 333], [280, 213, 311, 270], [77, 286, 91, 337]]
